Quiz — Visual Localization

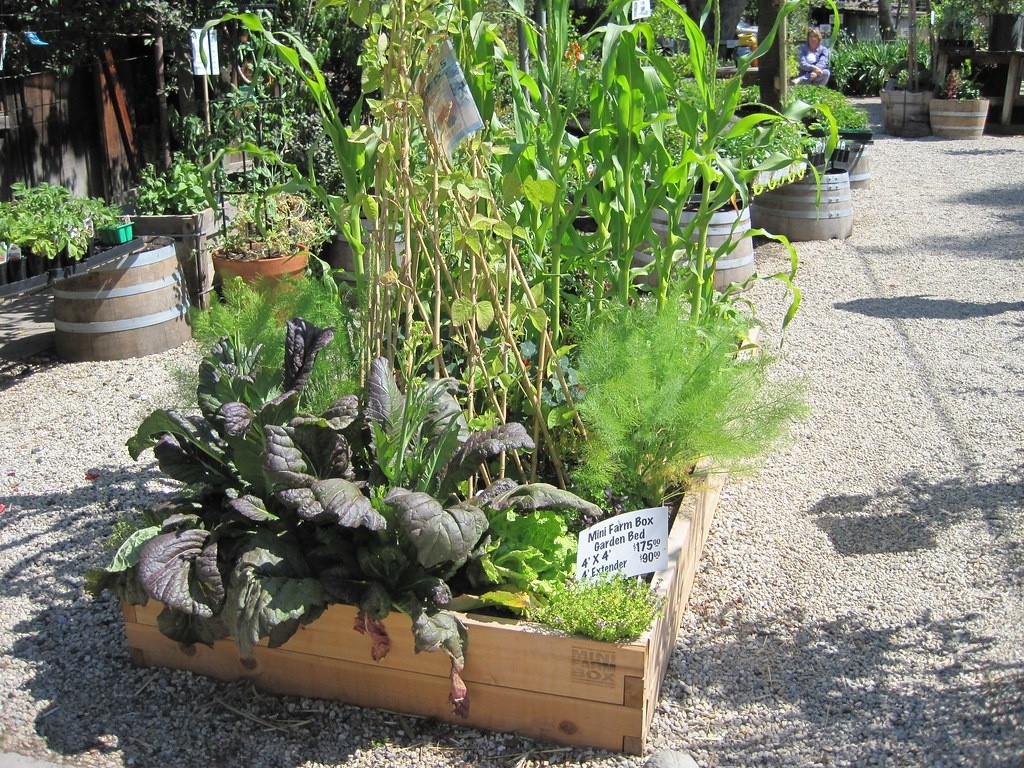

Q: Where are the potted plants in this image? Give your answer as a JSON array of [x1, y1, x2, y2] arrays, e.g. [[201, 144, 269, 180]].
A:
[[0, 0, 1024, 757]]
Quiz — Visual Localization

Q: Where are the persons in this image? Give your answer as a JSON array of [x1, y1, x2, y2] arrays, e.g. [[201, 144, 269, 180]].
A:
[[790, 29, 830, 87]]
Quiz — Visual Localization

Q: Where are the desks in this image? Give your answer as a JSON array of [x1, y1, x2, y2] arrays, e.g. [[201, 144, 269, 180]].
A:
[[933, 51, 1024, 125]]
